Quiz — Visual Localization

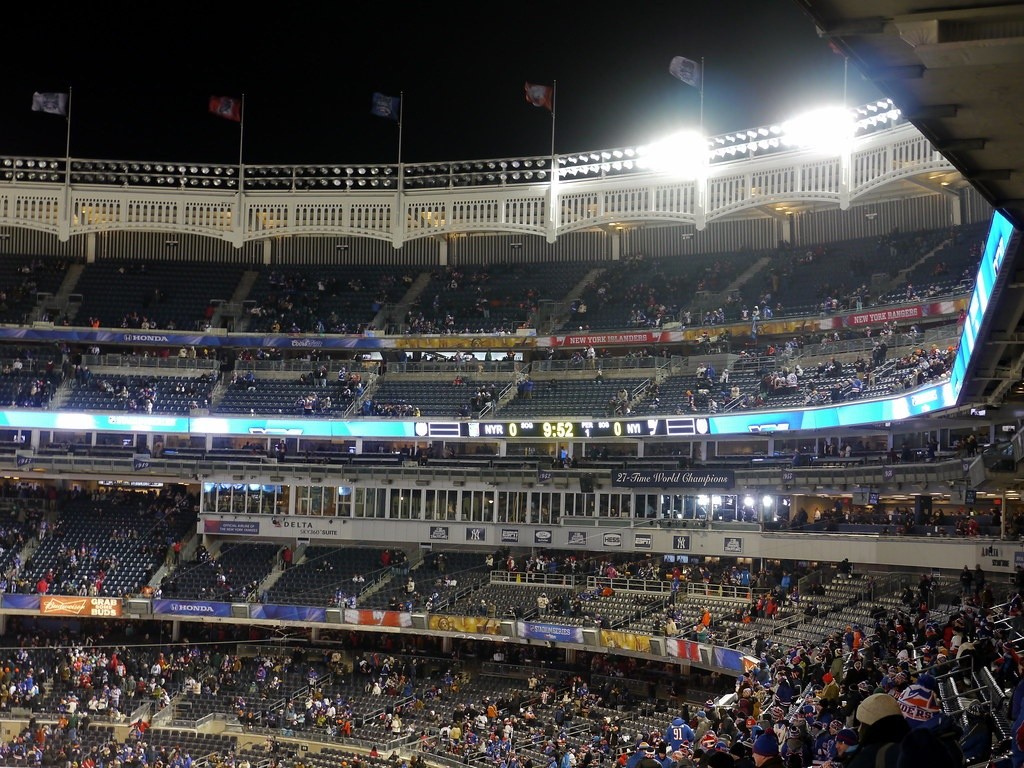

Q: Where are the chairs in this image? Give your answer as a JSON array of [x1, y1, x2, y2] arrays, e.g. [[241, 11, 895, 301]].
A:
[[0, 254, 1024, 768]]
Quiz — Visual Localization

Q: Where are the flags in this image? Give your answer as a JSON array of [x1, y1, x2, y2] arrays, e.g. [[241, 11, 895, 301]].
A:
[[370, 92, 401, 121], [524, 80, 554, 111], [669, 57, 704, 90], [832, 43, 845, 59], [32, 91, 70, 115], [209, 94, 242, 122]]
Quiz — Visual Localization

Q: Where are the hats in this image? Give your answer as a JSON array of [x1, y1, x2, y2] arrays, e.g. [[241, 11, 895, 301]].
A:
[[750, 625, 874, 738], [1002, 642, 1014, 650], [1019, 657, 1024, 667], [753, 728, 779, 757], [966, 699, 985, 716], [728, 742, 745, 758], [707, 751, 735, 768], [835, 729, 859, 746], [637, 672, 751, 755], [856, 693, 902, 725], [898, 674, 940, 728], [876, 564, 1008, 681]]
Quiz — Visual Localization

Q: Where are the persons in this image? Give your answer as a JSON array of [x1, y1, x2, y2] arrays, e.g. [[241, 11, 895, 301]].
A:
[[1, 339, 983, 429], [0, 220, 982, 339], [0, 610, 1024, 767], [0, 429, 1023, 609]]
